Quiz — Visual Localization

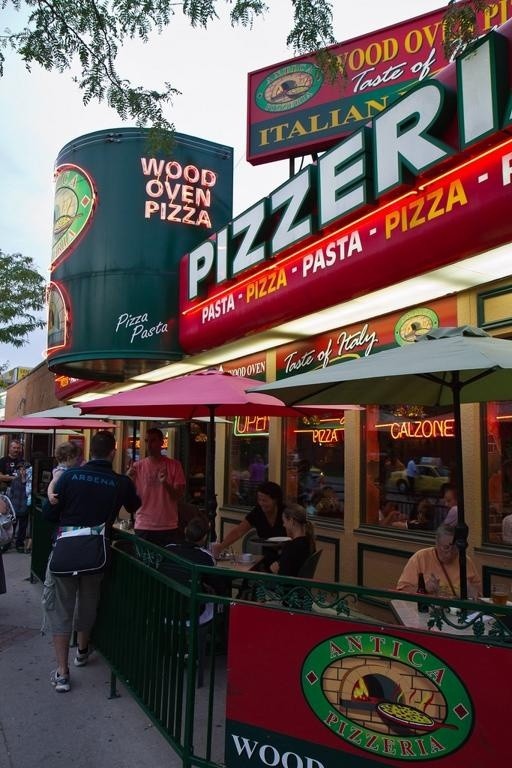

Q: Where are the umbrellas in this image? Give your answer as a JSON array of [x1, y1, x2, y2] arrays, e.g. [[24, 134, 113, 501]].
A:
[[245, 326, 512, 601]]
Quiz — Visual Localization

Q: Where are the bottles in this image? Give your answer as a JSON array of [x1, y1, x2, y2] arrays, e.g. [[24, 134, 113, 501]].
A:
[[417, 572, 429, 612]]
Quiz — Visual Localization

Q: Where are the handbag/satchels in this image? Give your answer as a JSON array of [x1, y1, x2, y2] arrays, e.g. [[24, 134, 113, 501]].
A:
[[48, 523, 109, 579]]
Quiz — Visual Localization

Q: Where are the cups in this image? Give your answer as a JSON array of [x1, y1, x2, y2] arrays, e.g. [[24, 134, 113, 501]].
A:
[[229, 552, 240, 570], [491, 583, 508, 607], [243, 554, 253, 561]]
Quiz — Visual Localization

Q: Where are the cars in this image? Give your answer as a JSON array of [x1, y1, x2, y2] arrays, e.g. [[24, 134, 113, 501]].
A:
[[388, 465, 449, 494]]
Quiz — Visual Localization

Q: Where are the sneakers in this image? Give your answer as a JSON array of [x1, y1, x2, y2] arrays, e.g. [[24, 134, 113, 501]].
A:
[[73, 643, 94, 667], [49, 669, 71, 693]]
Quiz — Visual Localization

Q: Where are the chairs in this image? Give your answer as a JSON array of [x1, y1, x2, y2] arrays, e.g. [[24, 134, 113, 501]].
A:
[[142, 549, 323, 689]]
